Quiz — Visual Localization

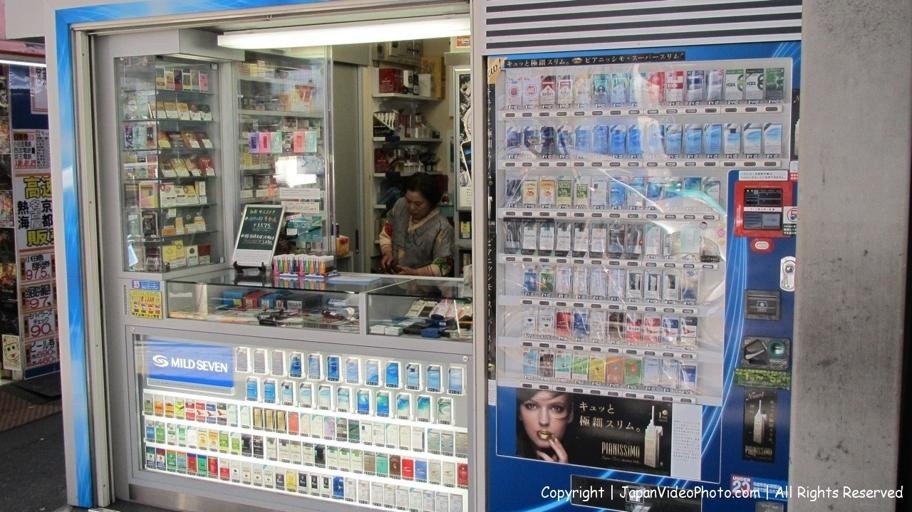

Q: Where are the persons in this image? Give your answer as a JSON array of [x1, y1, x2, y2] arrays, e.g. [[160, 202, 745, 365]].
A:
[[515, 388, 587, 465], [373, 171, 454, 316]]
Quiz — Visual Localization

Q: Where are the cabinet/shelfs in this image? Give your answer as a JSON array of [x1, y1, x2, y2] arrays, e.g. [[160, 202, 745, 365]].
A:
[[0, 63, 60, 381], [94, 29, 471, 512]]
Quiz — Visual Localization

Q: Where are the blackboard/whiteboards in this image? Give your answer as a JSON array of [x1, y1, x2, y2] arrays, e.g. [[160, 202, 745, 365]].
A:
[[235, 204, 286, 253]]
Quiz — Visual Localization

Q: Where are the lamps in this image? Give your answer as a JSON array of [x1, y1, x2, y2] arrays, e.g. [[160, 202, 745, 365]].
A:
[[0, 54, 46, 68], [217, 13, 471, 49]]
